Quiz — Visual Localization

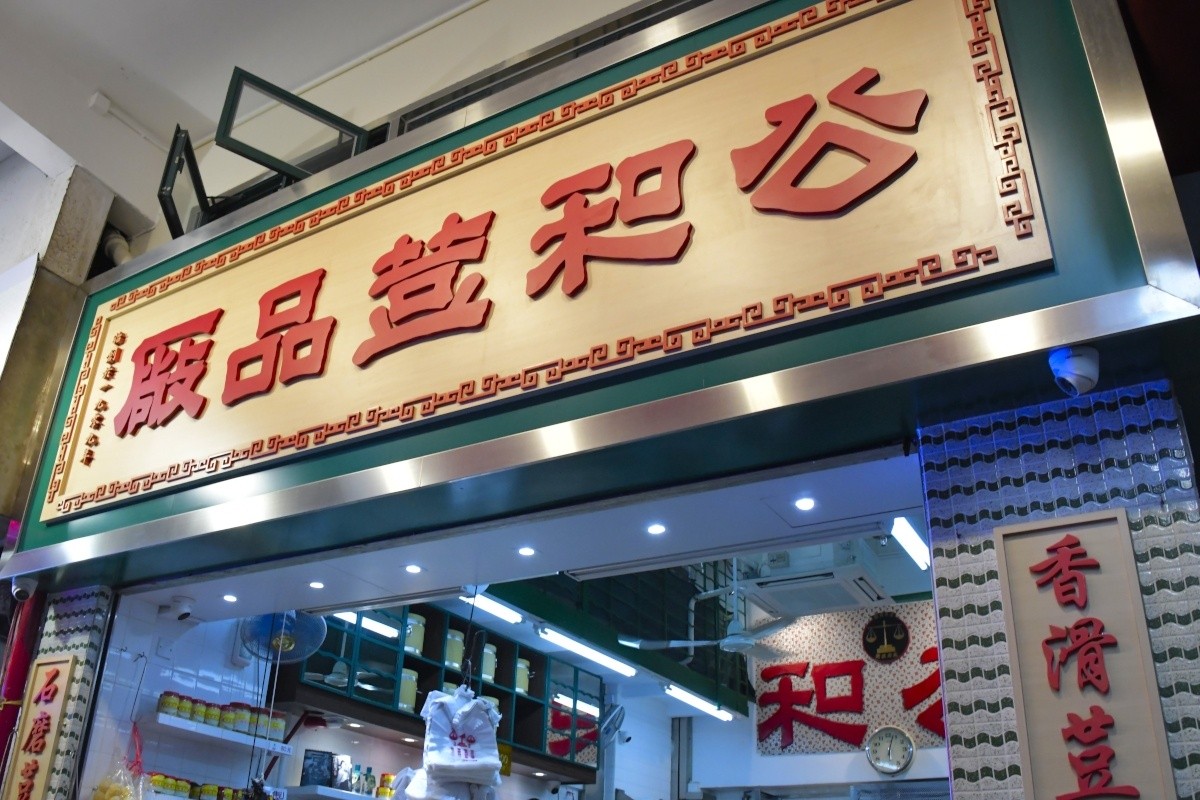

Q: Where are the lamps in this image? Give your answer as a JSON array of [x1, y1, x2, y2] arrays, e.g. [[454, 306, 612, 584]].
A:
[[305, 583, 489, 616], [534, 620, 638, 678], [459, 593, 527, 626], [661, 681, 735, 722], [333, 610, 402, 639], [565, 522, 889, 583], [891, 517, 930, 572]]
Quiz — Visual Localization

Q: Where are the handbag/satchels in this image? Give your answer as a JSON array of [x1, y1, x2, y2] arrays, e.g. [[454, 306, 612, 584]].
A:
[[92, 760, 156, 800]]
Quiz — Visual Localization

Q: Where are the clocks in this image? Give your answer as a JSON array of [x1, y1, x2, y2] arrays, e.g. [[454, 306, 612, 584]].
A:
[[865, 726, 916, 775]]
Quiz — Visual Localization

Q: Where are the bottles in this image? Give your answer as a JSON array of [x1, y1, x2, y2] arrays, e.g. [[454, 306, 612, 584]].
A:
[[364, 767, 372, 795], [352, 764, 360, 794]]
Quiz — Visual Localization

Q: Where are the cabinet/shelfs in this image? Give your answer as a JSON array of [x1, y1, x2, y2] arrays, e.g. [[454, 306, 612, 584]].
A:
[[268, 601, 607, 786], [132, 712, 293, 800]]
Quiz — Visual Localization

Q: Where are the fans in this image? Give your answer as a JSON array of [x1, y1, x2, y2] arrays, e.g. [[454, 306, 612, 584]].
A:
[[600, 703, 626, 746], [663, 557, 795, 663], [232, 611, 327, 667]]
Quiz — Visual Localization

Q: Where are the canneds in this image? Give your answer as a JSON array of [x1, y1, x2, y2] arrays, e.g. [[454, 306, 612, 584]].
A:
[[156, 691, 285, 744], [376, 773, 397, 800], [147, 772, 243, 800]]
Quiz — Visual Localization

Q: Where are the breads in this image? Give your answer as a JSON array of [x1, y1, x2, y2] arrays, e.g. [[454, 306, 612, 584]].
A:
[[94, 780, 133, 800]]
